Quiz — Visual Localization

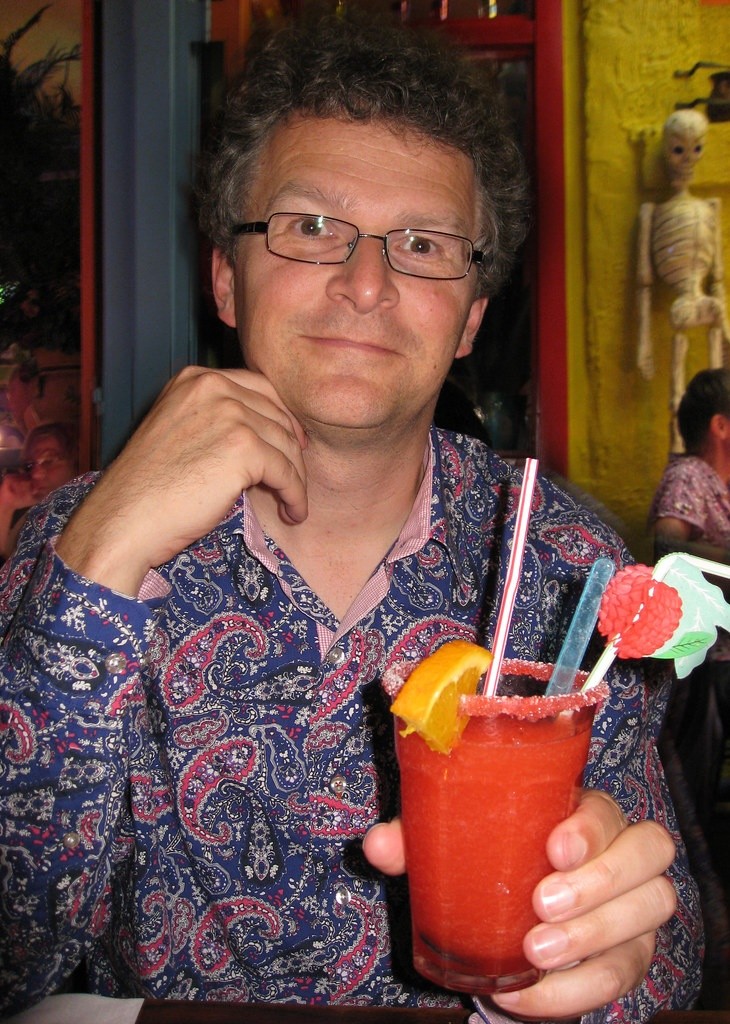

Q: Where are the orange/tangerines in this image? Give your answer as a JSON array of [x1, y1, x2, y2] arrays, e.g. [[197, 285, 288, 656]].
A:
[[389, 639, 495, 755]]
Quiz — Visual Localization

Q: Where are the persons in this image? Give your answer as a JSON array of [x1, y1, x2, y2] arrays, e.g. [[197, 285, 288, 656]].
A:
[[1, 286, 78, 564], [0, 24, 708, 1024], [647, 368, 730, 733]]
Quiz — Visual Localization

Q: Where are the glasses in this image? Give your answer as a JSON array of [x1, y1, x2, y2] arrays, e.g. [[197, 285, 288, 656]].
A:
[[231, 211, 484, 280]]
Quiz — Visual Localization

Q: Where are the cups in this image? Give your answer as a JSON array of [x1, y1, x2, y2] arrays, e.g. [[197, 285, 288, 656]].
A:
[[380, 658, 611, 995]]
